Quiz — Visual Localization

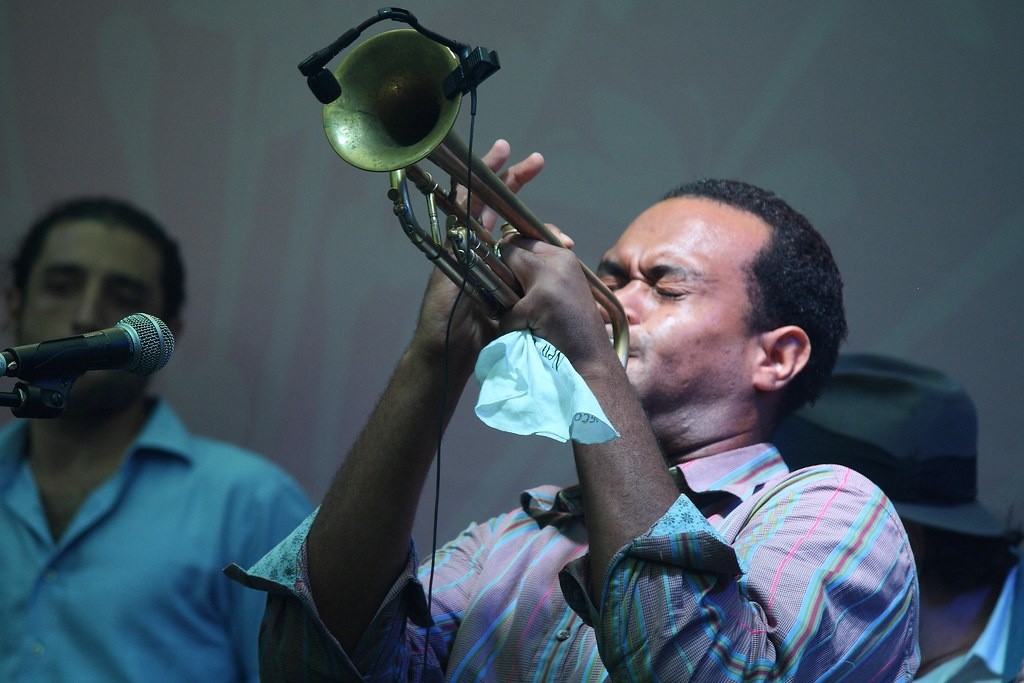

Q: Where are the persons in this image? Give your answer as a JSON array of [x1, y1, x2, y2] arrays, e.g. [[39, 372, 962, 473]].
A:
[[769, 352, 1024, 682], [0, 193, 314, 683], [221, 138, 920, 683]]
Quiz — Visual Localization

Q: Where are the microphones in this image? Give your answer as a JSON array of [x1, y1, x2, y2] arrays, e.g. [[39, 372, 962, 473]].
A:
[[0, 311, 176, 378], [297, 7, 464, 104]]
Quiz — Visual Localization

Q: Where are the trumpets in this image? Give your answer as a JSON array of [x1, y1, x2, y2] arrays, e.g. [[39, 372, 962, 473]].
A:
[[323, 28, 632, 384]]
